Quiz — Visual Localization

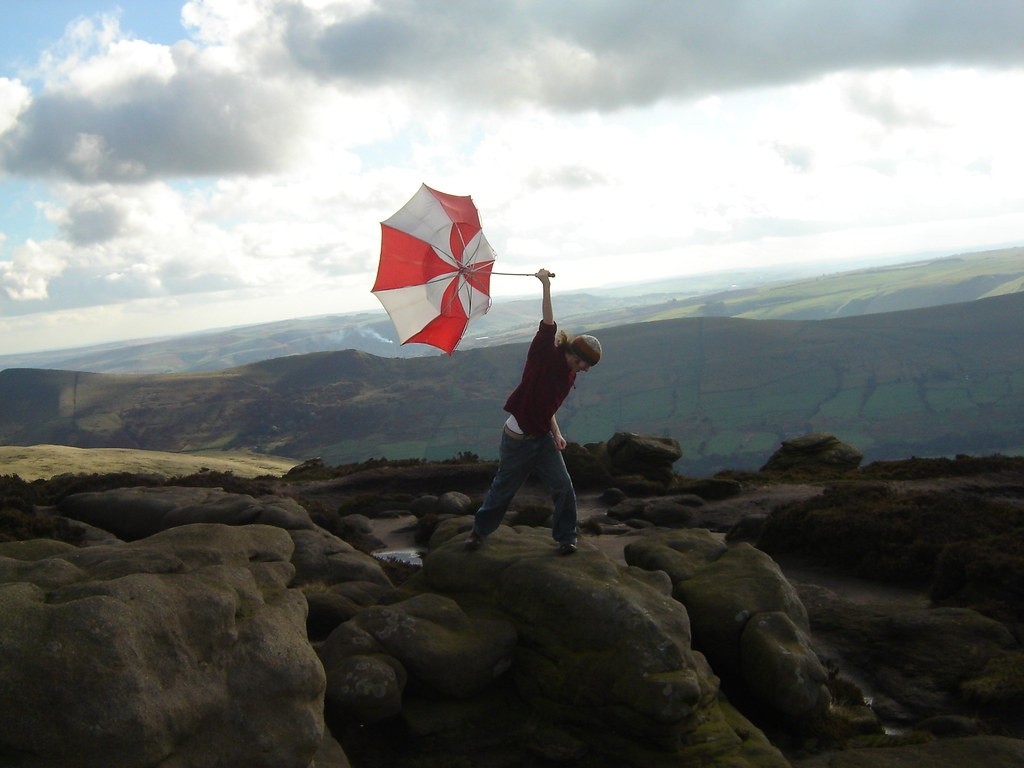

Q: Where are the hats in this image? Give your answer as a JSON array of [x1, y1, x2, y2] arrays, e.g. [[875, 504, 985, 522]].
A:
[[572, 334, 603, 366]]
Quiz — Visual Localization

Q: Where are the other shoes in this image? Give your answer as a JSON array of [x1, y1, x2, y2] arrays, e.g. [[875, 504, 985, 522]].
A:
[[559, 542, 577, 555], [466, 529, 483, 551]]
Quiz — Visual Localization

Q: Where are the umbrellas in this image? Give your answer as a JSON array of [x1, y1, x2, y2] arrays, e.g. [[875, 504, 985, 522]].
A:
[[369, 180, 557, 357]]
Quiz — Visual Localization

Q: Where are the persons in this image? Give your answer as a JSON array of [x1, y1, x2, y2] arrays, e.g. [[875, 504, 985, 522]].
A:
[[465, 267, 606, 555]]
